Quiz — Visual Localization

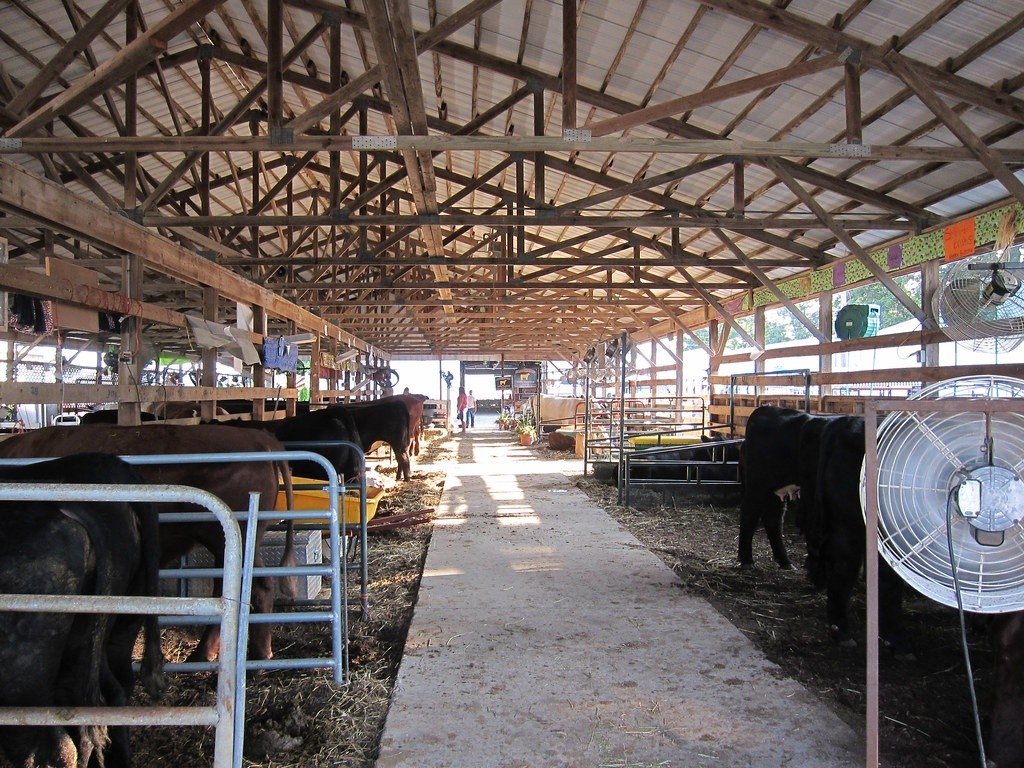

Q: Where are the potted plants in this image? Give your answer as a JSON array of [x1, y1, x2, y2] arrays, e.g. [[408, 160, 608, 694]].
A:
[[515, 423, 535, 445], [497, 417, 518, 430]]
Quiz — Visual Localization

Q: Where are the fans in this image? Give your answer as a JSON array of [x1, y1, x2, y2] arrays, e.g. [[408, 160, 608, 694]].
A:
[[932, 255, 1024, 353], [860, 374, 1024, 614]]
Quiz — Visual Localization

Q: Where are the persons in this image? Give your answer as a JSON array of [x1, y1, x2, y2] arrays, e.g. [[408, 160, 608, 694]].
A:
[[465, 390, 477, 428], [458, 387, 466, 433]]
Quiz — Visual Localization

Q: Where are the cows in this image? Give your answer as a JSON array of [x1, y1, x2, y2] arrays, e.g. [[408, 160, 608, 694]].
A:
[[739, 406, 905, 655], [0, 386, 430, 768], [522, 394, 610, 452], [613, 431, 739, 483]]
